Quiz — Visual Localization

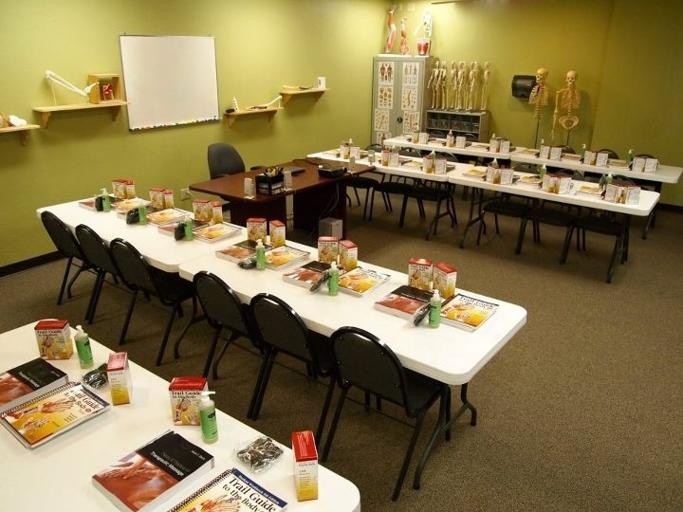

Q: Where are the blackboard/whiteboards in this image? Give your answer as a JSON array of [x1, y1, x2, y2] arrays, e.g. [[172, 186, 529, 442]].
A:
[[120, 36, 219, 131]]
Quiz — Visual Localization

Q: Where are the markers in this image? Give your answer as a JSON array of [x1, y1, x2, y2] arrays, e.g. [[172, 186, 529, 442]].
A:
[[134, 118, 216, 130]]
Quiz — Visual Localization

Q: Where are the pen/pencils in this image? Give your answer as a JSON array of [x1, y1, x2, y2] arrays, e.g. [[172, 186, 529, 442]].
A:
[[262, 166, 283, 177]]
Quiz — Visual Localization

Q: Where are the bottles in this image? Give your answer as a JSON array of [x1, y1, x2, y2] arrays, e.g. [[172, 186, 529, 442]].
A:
[[197, 390, 220, 445], [328, 261, 339, 296], [428, 290, 441, 327], [100, 186, 197, 240], [72, 325, 95, 370], [339, 126, 634, 203], [254, 239, 266, 270]]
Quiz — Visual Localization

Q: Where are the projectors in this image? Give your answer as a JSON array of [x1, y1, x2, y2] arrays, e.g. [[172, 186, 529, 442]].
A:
[[318, 165, 347, 178]]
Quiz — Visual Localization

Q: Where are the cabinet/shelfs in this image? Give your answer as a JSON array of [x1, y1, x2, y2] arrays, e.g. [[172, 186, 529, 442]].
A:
[[371, 54, 437, 148], [424, 108, 490, 141]]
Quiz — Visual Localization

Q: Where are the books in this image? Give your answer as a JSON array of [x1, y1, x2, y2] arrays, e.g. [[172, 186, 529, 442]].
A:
[[77, 192, 310, 272], [323, 139, 641, 204], [439, 292, 501, 335], [1, 357, 70, 413], [338, 265, 392, 298], [1, 378, 114, 451], [373, 284, 435, 320], [90, 429, 216, 512], [282, 259, 344, 294], [167, 466, 291, 512]]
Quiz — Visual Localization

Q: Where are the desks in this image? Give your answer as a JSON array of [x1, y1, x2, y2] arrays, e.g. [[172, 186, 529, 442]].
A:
[[0, 318, 361, 512], [189, 158, 376, 236]]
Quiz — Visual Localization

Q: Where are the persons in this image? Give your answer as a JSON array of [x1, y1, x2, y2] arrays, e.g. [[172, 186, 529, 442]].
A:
[[378, 63, 418, 84]]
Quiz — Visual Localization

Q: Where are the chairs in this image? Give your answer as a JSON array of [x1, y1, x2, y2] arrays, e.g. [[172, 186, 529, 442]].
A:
[[208, 143, 267, 179]]
